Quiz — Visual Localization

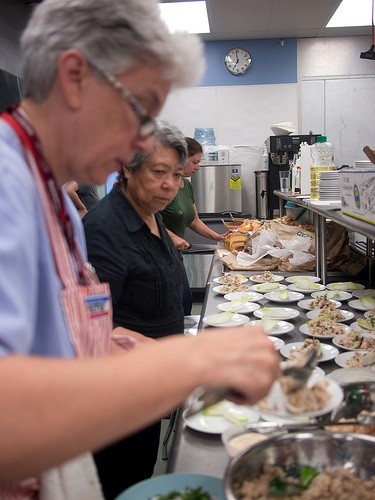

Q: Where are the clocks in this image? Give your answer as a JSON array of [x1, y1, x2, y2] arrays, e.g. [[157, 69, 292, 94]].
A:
[[224, 47, 251, 76]]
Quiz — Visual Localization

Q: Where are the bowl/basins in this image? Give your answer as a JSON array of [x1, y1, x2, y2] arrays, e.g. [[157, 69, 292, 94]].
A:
[[224, 430, 375, 499], [114, 471, 224, 500]]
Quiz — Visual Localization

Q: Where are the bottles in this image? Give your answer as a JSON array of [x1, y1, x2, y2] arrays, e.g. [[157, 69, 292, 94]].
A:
[[294, 166, 301, 194], [311, 136, 335, 199]]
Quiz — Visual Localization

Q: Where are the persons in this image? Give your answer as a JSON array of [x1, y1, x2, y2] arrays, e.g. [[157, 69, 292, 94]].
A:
[[158, 136, 231, 251], [65, 177, 101, 220], [0, 0, 281, 500], [82, 120, 193, 339]]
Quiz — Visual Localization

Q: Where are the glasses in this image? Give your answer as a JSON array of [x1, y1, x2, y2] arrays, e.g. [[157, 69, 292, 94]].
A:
[[86, 58, 159, 140]]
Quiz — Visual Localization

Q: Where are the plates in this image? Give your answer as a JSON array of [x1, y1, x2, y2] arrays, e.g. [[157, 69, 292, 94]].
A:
[[181, 274, 375, 433], [310, 160, 375, 210]]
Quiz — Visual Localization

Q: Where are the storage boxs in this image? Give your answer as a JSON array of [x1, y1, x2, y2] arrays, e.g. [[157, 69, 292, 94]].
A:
[[338, 170, 375, 225], [200, 147, 229, 165]]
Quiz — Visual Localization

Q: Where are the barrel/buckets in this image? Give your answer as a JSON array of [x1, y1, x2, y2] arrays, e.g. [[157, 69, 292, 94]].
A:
[[254, 170, 271, 219]]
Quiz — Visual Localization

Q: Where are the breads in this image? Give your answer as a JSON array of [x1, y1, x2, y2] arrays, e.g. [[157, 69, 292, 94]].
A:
[[224, 215, 313, 252]]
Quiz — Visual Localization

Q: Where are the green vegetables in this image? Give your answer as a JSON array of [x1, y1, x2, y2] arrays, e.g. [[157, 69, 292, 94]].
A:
[[266, 462, 319, 497], [343, 385, 363, 404], [156, 487, 213, 500]]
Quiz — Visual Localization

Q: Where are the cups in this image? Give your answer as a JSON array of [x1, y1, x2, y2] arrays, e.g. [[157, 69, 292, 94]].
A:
[[279, 171, 289, 192]]
